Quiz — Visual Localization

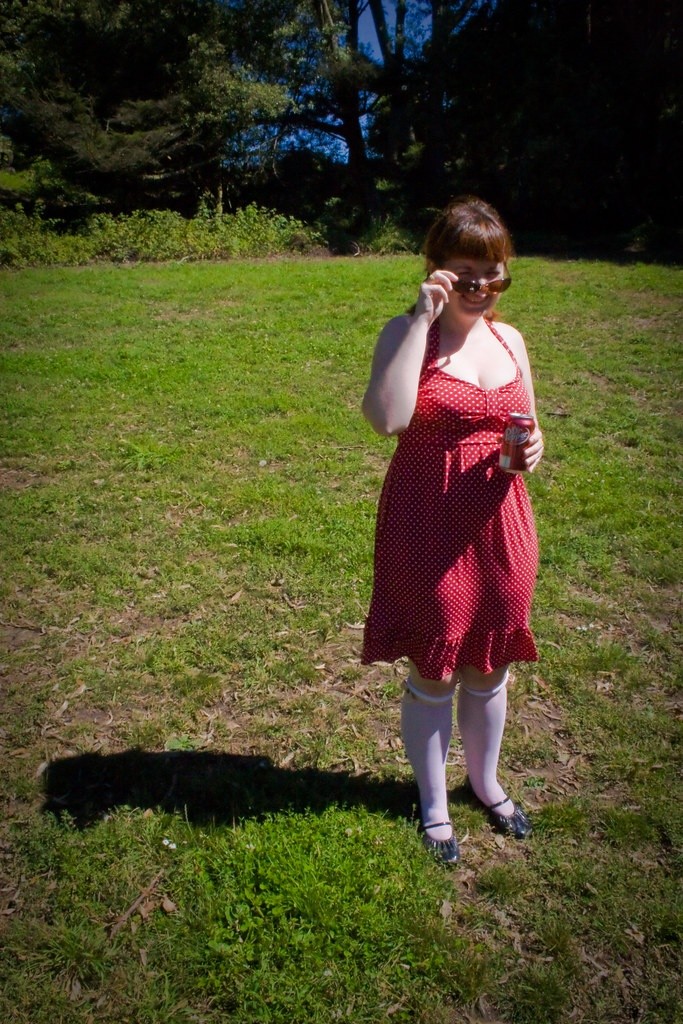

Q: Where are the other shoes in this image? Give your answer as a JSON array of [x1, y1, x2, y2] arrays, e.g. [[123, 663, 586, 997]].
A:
[[418, 821, 460, 865], [464, 773, 529, 839]]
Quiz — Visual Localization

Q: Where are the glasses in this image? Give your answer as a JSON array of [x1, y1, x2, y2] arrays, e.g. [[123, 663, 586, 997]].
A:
[[453, 262, 513, 296]]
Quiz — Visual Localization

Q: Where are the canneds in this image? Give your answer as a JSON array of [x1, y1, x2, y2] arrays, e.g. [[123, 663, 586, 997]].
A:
[[499, 413, 535, 473]]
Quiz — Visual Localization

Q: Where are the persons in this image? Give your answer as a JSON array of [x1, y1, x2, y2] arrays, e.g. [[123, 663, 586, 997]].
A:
[[360, 199, 543, 865]]
